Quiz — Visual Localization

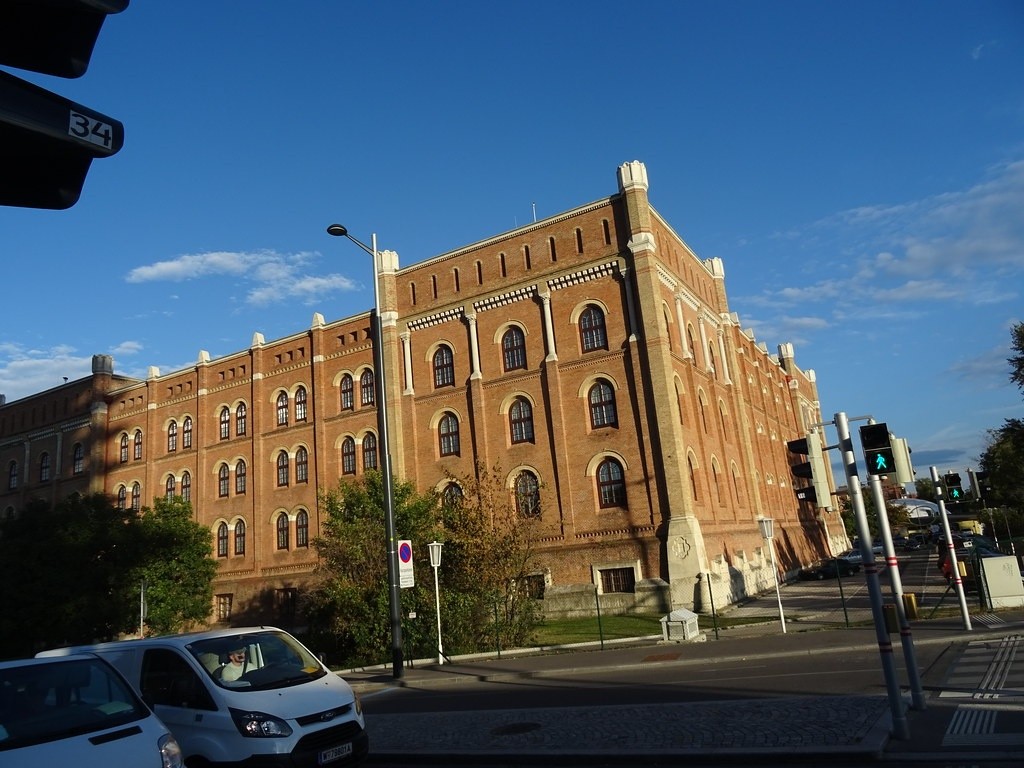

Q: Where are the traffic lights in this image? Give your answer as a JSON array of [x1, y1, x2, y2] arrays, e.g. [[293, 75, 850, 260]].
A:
[[943, 472, 964, 501], [966, 468, 992, 500], [858, 422, 897, 477], [0, 0, 128, 214], [787, 430, 832, 508]]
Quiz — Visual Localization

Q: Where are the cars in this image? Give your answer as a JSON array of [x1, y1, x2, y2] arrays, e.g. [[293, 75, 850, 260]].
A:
[[871, 542, 884, 554], [914, 535, 928, 545], [936, 532, 1007, 595], [837, 548, 863, 565], [798, 557, 860, 582], [904, 538, 920, 552]]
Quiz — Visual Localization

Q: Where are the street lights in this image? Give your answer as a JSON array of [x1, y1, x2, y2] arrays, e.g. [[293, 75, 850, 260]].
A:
[[427, 541, 444, 665], [325, 223, 404, 678], [757, 518, 788, 634]]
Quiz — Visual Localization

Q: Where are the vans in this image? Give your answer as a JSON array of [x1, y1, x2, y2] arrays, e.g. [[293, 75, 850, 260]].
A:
[[0, 651, 185, 768], [36, 625, 367, 768]]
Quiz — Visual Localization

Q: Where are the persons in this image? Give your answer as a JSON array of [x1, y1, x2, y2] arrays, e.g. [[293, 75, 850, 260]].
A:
[[222, 649, 258, 684]]
